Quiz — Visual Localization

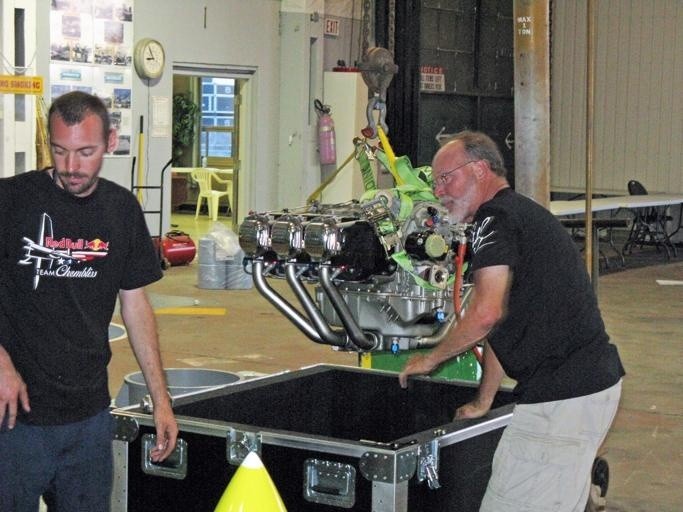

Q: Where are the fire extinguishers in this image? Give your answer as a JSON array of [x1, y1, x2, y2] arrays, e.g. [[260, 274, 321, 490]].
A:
[[314, 98, 336, 165]]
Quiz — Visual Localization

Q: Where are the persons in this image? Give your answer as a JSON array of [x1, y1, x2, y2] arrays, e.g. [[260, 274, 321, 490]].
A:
[[0, 90, 178, 511], [48, 0, 132, 67], [395, 132, 625, 509]]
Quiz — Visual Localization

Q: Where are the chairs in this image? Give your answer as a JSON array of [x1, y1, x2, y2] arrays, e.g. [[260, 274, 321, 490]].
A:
[[190, 168, 234, 222], [625, 180, 674, 252]]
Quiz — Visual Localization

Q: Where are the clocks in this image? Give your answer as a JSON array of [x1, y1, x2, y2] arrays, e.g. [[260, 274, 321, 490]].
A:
[[133, 37, 167, 81]]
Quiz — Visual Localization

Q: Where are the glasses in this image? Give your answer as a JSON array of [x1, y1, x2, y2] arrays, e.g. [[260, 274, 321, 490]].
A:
[[432, 160, 476, 188]]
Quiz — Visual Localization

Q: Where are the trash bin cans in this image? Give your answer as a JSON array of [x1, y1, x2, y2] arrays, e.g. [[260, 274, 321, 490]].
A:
[[115, 368, 245, 409]]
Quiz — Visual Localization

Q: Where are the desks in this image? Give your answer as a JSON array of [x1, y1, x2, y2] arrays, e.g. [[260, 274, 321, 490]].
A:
[[548, 185, 683, 269], [171, 166, 233, 216]]
